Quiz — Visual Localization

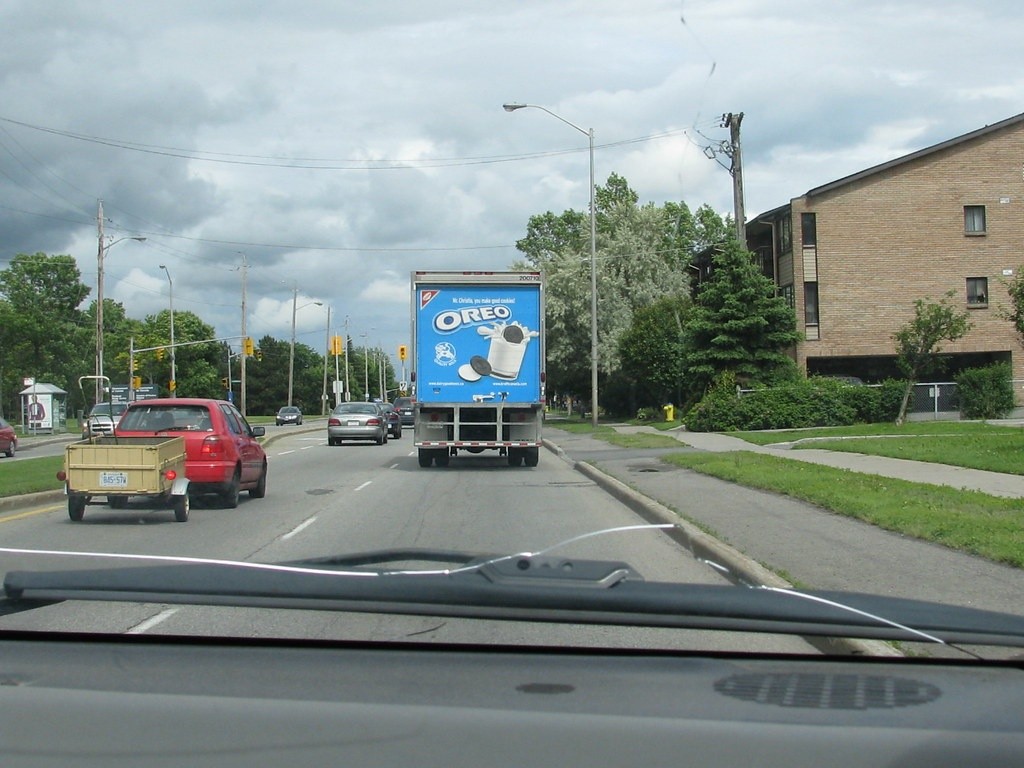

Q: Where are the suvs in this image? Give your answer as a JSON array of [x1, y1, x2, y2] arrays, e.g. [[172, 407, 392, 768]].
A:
[[82, 401, 136, 441], [393, 396, 415, 425]]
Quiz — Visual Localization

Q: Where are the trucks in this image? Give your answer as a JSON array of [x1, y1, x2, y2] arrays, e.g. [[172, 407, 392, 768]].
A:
[[408, 270, 548, 468]]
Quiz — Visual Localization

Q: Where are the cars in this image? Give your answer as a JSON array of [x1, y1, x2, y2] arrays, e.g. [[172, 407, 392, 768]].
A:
[[362, 402, 403, 439], [327, 401, 391, 446], [113, 397, 269, 508], [0, 416, 18, 457], [275, 407, 303, 426]]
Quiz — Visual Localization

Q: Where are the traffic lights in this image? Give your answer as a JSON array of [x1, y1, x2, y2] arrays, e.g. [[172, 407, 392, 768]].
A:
[[222, 378, 228, 388], [132, 359, 139, 372], [157, 349, 164, 362], [399, 346, 407, 359], [257, 350, 263, 363], [167, 379, 175, 393]]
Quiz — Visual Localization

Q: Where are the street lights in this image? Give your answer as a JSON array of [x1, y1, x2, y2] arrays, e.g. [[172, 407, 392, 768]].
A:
[[159, 265, 175, 397], [94, 236, 148, 403], [346, 332, 367, 402], [502, 101, 600, 430]]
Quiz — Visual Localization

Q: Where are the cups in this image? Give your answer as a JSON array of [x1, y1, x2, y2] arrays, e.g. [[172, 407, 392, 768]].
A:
[[487, 337, 526, 381]]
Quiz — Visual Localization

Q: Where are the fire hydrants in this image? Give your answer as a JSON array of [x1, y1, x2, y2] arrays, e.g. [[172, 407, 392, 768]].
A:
[[663, 402, 675, 422], [287, 300, 323, 407]]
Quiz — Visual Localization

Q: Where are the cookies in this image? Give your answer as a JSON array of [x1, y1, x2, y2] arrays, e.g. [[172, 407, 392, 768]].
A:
[[458, 355, 492, 382], [502, 325, 524, 344]]
